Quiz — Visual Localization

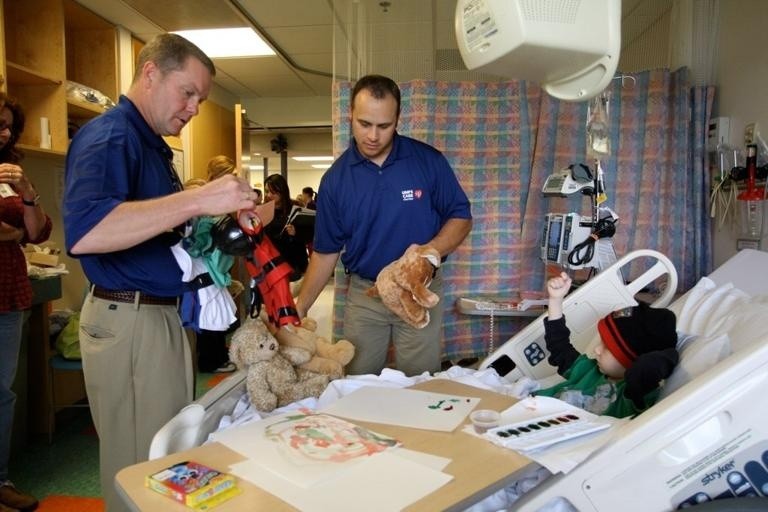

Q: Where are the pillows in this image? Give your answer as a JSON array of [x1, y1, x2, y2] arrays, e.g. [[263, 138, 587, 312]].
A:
[[658, 329, 732, 400]]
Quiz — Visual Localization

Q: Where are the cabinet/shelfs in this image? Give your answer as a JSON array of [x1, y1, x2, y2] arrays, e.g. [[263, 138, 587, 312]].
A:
[[0, 0, 118, 151], [189, 98, 236, 184], [132, 36, 180, 140]]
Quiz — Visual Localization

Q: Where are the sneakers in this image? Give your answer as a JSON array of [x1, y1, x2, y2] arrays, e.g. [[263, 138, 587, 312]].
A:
[[212, 361, 235, 373], [0, 479, 38, 512]]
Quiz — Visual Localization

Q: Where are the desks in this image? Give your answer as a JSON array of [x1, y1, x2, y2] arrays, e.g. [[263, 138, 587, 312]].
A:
[[451, 297, 549, 318], [633, 291, 686, 308], [111, 376, 533, 512], [0, 253, 63, 453]]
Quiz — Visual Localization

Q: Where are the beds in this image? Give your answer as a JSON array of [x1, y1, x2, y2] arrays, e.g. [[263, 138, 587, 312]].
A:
[[148, 243, 768, 512]]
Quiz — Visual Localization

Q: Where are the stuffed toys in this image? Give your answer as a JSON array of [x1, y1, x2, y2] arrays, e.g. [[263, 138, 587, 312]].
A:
[[362, 242, 441, 329], [232, 314, 331, 415], [257, 315, 356, 375]]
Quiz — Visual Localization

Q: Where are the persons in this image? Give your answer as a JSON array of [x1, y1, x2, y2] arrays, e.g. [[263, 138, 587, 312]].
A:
[[0, 90, 54, 511], [526, 273, 679, 422], [294, 72, 474, 380], [166, 154, 321, 374], [60, 31, 261, 511]]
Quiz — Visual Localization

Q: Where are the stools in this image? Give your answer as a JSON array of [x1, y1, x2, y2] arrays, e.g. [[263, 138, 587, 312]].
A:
[[44, 354, 91, 434]]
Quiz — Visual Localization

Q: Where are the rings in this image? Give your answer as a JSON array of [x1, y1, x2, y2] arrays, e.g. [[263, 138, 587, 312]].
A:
[[11, 172, 13, 177]]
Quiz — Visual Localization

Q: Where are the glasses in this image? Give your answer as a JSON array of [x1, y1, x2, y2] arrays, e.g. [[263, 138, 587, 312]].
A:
[[0, 121, 17, 134]]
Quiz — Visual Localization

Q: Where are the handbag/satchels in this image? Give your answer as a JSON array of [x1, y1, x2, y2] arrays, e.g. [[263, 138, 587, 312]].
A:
[[56, 312, 82, 359]]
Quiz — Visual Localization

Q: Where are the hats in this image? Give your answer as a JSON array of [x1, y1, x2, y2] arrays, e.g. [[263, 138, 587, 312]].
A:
[[598, 305, 677, 370]]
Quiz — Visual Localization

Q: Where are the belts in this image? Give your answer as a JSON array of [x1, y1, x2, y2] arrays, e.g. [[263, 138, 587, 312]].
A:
[[89, 283, 183, 306]]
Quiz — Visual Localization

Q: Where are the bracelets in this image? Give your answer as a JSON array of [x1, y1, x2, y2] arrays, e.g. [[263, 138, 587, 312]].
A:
[[22, 194, 41, 206]]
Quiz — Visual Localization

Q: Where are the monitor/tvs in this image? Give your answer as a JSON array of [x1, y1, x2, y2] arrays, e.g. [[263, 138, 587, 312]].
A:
[[454, 2, 621, 103]]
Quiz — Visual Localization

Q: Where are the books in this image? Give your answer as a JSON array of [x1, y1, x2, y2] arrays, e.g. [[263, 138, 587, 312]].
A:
[[29, 252, 59, 267]]
